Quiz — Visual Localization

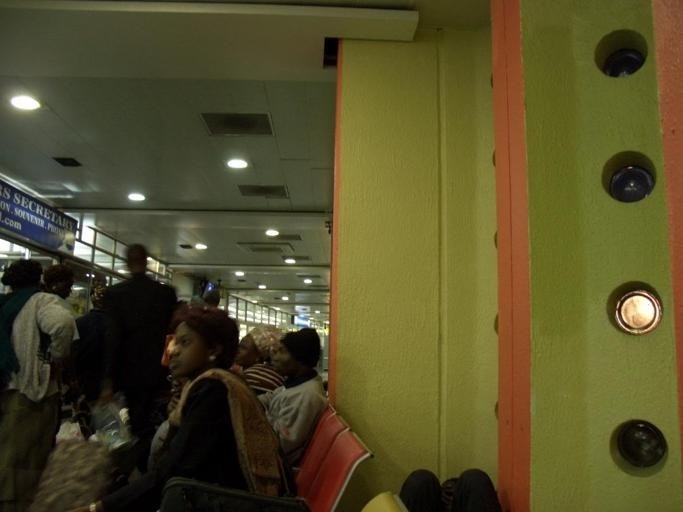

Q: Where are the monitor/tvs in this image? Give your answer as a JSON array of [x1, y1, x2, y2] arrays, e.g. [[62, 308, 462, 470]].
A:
[[200, 280, 216, 300]]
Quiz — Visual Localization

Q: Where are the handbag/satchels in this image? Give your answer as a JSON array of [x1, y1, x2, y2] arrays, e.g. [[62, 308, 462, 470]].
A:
[[35, 297, 55, 362]]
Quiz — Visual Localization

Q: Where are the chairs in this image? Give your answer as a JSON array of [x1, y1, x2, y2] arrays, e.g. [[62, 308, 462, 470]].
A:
[[157, 402, 410, 511]]
